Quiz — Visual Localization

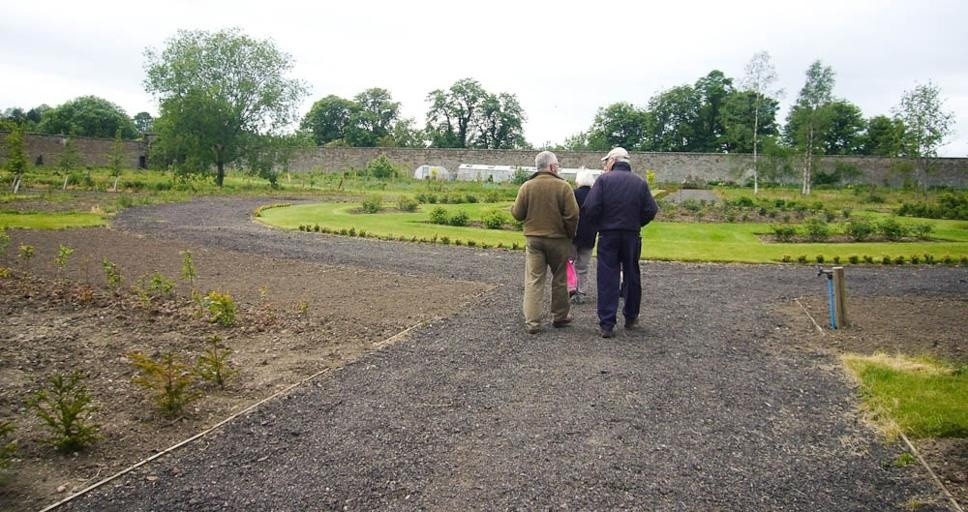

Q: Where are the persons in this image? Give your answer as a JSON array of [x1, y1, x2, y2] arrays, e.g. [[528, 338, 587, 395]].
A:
[[585, 147, 657, 338], [510, 150, 580, 334], [569, 169, 597, 304]]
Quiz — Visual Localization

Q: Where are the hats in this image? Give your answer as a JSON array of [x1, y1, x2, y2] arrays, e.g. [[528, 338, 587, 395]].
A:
[[601, 147, 630, 161]]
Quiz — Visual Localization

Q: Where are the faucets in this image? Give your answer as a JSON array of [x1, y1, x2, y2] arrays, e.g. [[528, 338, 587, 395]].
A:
[[815, 266, 833, 281]]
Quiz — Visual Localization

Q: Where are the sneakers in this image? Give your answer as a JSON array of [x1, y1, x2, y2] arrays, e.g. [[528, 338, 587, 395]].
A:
[[624, 317, 640, 330], [569, 290, 586, 305], [552, 313, 574, 328], [600, 327, 613, 338], [528, 329, 540, 334]]
